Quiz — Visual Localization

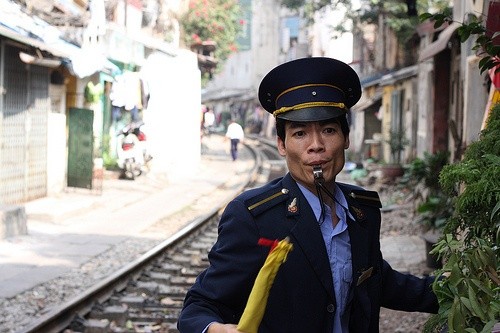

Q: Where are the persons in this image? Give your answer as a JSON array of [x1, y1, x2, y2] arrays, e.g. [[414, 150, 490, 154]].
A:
[[226, 119, 244, 161], [177, 57, 455, 333]]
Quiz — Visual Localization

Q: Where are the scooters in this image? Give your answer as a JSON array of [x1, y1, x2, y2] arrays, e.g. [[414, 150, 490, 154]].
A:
[[114, 121, 154, 181]]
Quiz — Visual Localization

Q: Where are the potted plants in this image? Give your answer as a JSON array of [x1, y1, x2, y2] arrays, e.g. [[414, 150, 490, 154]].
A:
[[380, 128, 409, 178]]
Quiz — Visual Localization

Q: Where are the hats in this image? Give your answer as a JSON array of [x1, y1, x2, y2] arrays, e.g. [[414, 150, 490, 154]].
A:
[[258, 57, 362, 121]]
